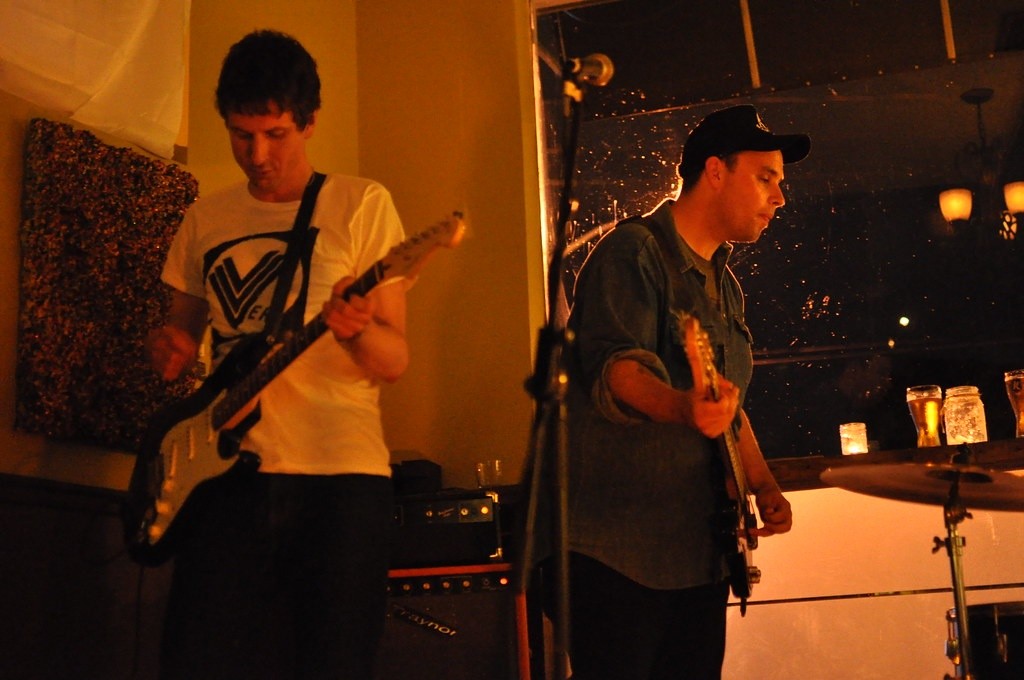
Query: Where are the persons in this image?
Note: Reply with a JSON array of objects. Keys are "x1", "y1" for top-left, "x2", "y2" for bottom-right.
[
  {"x1": 148, "y1": 29, "x2": 422, "y2": 679},
  {"x1": 542, "y1": 103, "x2": 809, "y2": 680}
]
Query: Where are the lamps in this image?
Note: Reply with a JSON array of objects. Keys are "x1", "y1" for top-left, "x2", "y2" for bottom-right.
[{"x1": 937, "y1": 86, "x2": 1024, "y2": 245}]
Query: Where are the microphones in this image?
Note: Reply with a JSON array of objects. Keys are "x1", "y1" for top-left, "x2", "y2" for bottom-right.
[{"x1": 563, "y1": 54, "x2": 614, "y2": 86}]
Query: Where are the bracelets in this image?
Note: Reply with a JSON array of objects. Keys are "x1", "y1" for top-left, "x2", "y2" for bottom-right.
[{"x1": 336, "y1": 324, "x2": 368, "y2": 342}]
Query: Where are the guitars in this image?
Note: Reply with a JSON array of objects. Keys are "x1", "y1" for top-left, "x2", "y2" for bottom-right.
[
  {"x1": 143, "y1": 208, "x2": 466, "y2": 568},
  {"x1": 666, "y1": 305, "x2": 761, "y2": 599}
]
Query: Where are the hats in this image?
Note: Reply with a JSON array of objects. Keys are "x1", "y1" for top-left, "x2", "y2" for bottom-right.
[{"x1": 683, "y1": 105, "x2": 813, "y2": 178}]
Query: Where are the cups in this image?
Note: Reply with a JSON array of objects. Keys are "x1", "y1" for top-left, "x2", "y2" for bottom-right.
[
  {"x1": 942, "y1": 386, "x2": 988, "y2": 445},
  {"x1": 471, "y1": 459, "x2": 503, "y2": 488},
  {"x1": 906, "y1": 384, "x2": 943, "y2": 447},
  {"x1": 838, "y1": 422, "x2": 869, "y2": 455},
  {"x1": 1005, "y1": 370, "x2": 1024, "y2": 438}
]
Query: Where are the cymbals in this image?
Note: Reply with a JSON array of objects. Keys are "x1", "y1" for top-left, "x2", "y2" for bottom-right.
[{"x1": 819, "y1": 461, "x2": 1024, "y2": 514}]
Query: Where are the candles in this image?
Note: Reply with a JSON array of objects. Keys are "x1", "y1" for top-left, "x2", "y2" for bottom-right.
[{"x1": 838, "y1": 422, "x2": 868, "y2": 456}]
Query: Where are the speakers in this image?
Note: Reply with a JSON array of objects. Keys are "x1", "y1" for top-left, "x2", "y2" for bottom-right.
[{"x1": 374, "y1": 563, "x2": 532, "y2": 680}]
